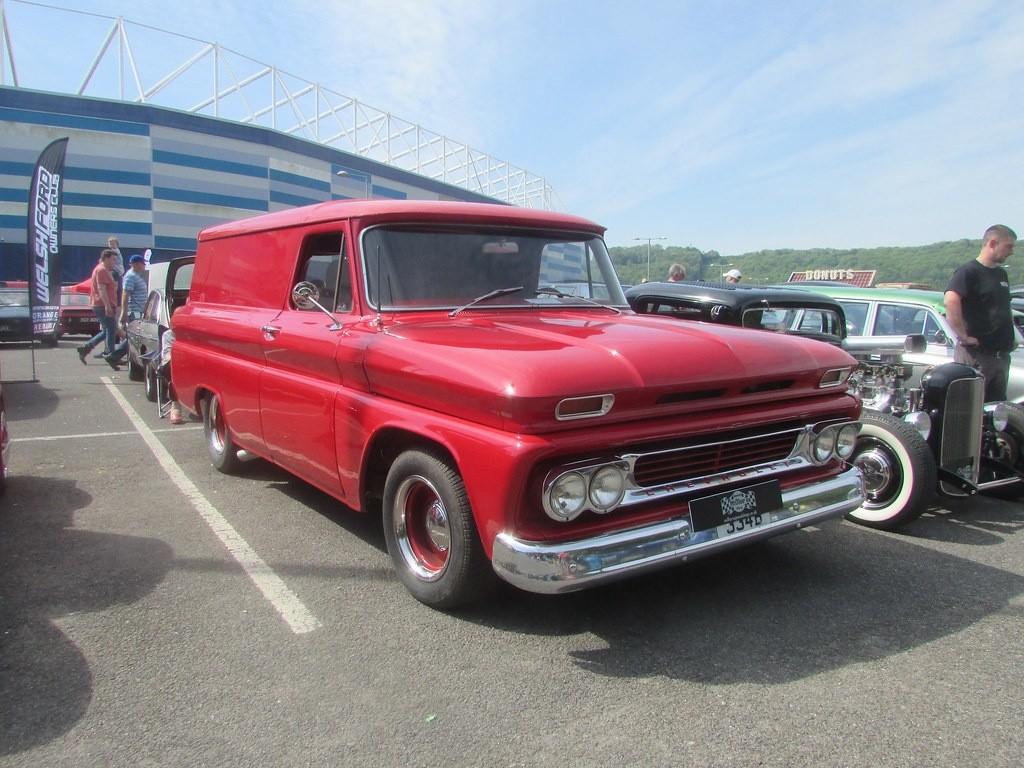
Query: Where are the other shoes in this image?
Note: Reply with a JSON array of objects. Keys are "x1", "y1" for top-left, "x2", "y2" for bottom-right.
[
  {"x1": 77, "y1": 346, "x2": 88, "y2": 364},
  {"x1": 170, "y1": 408, "x2": 182, "y2": 423},
  {"x1": 93, "y1": 351, "x2": 109, "y2": 358},
  {"x1": 117, "y1": 358, "x2": 127, "y2": 365},
  {"x1": 105, "y1": 356, "x2": 121, "y2": 370}
]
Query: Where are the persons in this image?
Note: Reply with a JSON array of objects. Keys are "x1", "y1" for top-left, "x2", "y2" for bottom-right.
[
  {"x1": 943, "y1": 224, "x2": 1017, "y2": 403},
  {"x1": 641, "y1": 278, "x2": 648, "y2": 283},
  {"x1": 76, "y1": 235, "x2": 149, "y2": 371},
  {"x1": 722, "y1": 269, "x2": 741, "y2": 283},
  {"x1": 667, "y1": 263, "x2": 686, "y2": 282},
  {"x1": 158, "y1": 330, "x2": 183, "y2": 424}
]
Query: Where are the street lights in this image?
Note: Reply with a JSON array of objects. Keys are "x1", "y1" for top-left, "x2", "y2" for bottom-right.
[
  {"x1": 710, "y1": 264, "x2": 735, "y2": 284},
  {"x1": 336, "y1": 171, "x2": 369, "y2": 199},
  {"x1": 749, "y1": 278, "x2": 769, "y2": 284},
  {"x1": 632, "y1": 236, "x2": 668, "y2": 281}
]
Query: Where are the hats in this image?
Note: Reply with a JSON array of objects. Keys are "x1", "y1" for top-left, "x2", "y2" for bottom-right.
[
  {"x1": 130, "y1": 255, "x2": 148, "y2": 263},
  {"x1": 723, "y1": 270, "x2": 742, "y2": 281}
]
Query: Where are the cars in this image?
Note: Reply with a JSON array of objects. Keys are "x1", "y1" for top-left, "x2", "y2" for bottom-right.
[
  {"x1": 126, "y1": 200, "x2": 1024, "y2": 607},
  {"x1": 0, "y1": 287, "x2": 32, "y2": 342},
  {"x1": 59, "y1": 293, "x2": 101, "y2": 339}
]
